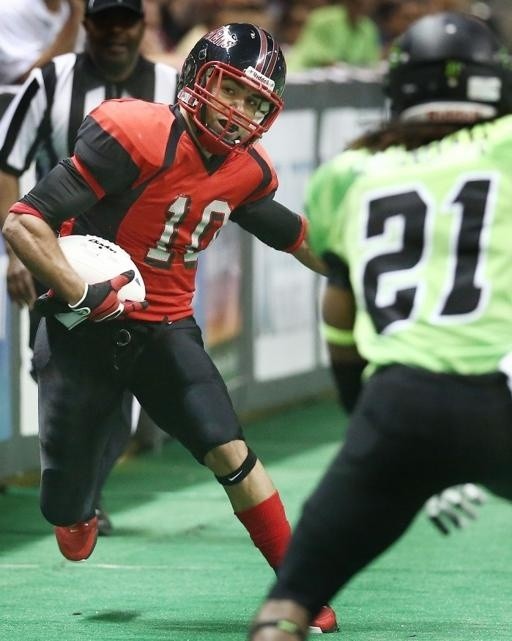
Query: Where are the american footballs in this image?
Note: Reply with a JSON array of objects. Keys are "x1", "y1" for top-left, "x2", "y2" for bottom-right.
[{"x1": 57, "y1": 234, "x2": 146, "y2": 303}]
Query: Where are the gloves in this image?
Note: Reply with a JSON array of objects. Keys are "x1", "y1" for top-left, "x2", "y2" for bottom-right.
[
  {"x1": 424, "y1": 483, "x2": 485, "y2": 536},
  {"x1": 68, "y1": 270, "x2": 149, "y2": 323}
]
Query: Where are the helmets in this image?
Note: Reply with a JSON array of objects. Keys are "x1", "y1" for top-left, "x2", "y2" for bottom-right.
[
  {"x1": 382, "y1": 11, "x2": 503, "y2": 120},
  {"x1": 177, "y1": 23, "x2": 287, "y2": 114},
  {"x1": 85, "y1": 0, "x2": 144, "y2": 17}
]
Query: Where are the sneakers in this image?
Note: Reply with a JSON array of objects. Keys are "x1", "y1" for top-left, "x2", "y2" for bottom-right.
[
  {"x1": 53, "y1": 506, "x2": 112, "y2": 562},
  {"x1": 248, "y1": 598, "x2": 338, "y2": 641}
]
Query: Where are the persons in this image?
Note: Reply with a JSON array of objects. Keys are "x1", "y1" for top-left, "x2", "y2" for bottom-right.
[
  {"x1": 0, "y1": 0, "x2": 511, "y2": 640},
  {"x1": 1, "y1": 2, "x2": 87, "y2": 85},
  {"x1": 249, "y1": 11, "x2": 508, "y2": 641},
  {"x1": 3, "y1": 22, "x2": 338, "y2": 635},
  {"x1": 141, "y1": 1, "x2": 435, "y2": 78}
]
